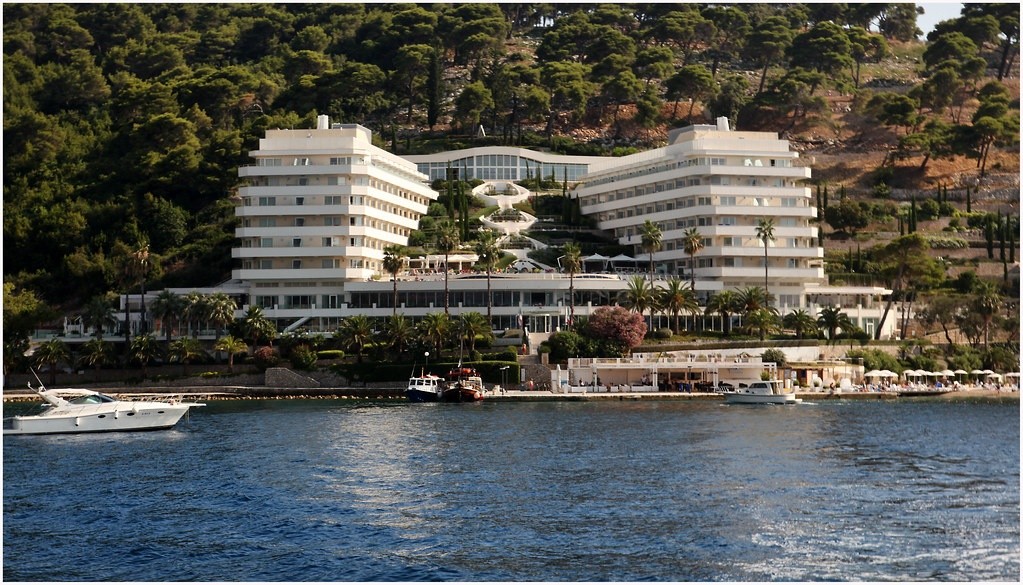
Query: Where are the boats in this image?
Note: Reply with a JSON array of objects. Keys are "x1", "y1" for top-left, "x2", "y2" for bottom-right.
[
  {"x1": 716, "y1": 381, "x2": 803, "y2": 404},
  {"x1": 404, "y1": 365, "x2": 446, "y2": 401},
  {"x1": 444, "y1": 336, "x2": 486, "y2": 402},
  {"x1": 12, "y1": 367, "x2": 206, "y2": 432}
]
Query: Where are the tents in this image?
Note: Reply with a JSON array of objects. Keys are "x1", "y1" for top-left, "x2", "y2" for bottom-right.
[{"x1": 582, "y1": 253, "x2": 636, "y2": 273}]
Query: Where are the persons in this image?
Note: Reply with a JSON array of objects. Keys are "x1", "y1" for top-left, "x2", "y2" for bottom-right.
[
  {"x1": 936, "y1": 380, "x2": 942, "y2": 387},
  {"x1": 861, "y1": 378, "x2": 882, "y2": 392},
  {"x1": 521, "y1": 343, "x2": 526, "y2": 356},
  {"x1": 641, "y1": 375, "x2": 649, "y2": 385},
  {"x1": 529, "y1": 378, "x2": 534, "y2": 391}
]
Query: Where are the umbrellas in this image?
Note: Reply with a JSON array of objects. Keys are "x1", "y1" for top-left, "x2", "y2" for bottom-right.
[
  {"x1": 987, "y1": 372, "x2": 1020, "y2": 385},
  {"x1": 863, "y1": 369, "x2": 899, "y2": 384},
  {"x1": 903, "y1": 369, "x2": 955, "y2": 385},
  {"x1": 982, "y1": 370, "x2": 994, "y2": 374},
  {"x1": 971, "y1": 369, "x2": 983, "y2": 385},
  {"x1": 954, "y1": 369, "x2": 967, "y2": 382}
]
